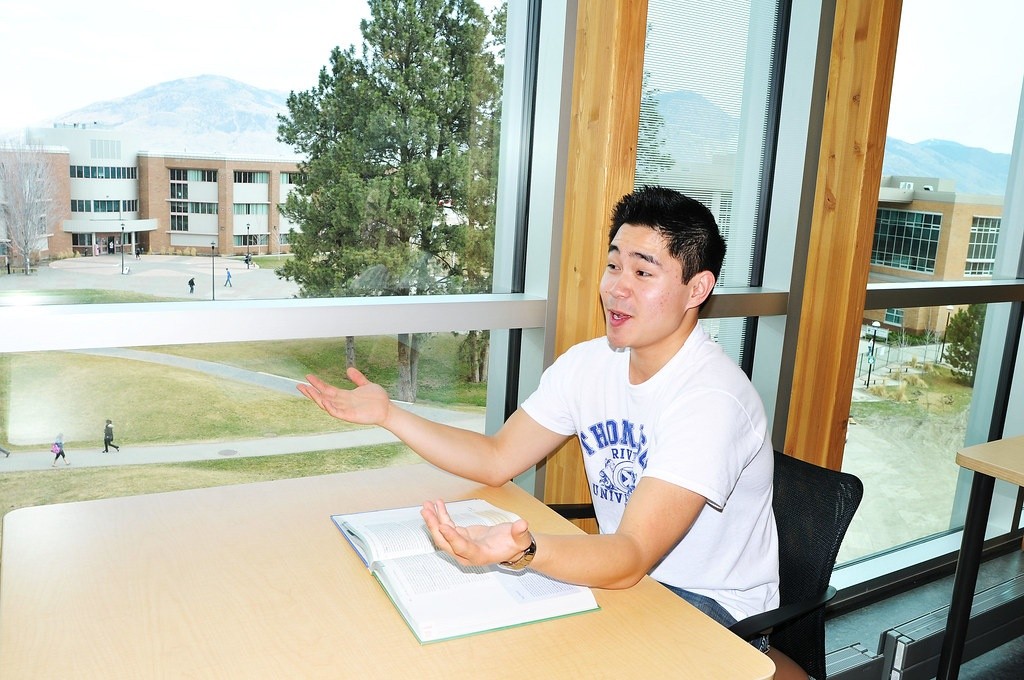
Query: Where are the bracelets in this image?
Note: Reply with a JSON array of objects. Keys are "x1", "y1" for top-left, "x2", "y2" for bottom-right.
[{"x1": 497, "y1": 531, "x2": 537, "y2": 571}]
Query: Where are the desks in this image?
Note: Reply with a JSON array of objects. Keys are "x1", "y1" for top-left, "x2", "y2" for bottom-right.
[
  {"x1": 0, "y1": 454, "x2": 784, "y2": 679},
  {"x1": 936, "y1": 435, "x2": 1024, "y2": 680}
]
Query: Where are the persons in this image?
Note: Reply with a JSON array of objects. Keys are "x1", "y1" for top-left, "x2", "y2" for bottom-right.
[
  {"x1": 52, "y1": 433, "x2": 70, "y2": 466},
  {"x1": 296, "y1": 184, "x2": 779, "y2": 653},
  {"x1": 224, "y1": 268, "x2": 232, "y2": 287},
  {"x1": 245, "y1": 252, "x2": 251, "y2": 264},
  {"x1": 103, "y1": 420, "x2": 119, "y2": 453},
  {"x1": 109, "y1": 240, "x2": 114, "y2": 254},
  {"x1": 136, "y1": 246, "x2": 143, "y2": 258},
  {"x1": 0, "y1": 447, "x2": 10, "y2": 457},
  {"x1": 867, "y1": 338, "x2": 873, "y2": 356},
  {"x1": 279, "y1": 268, "x2": 289, "y2": 281},
  {"x1": 188, "y1": 278, "x2": 195, "y2": 293}
]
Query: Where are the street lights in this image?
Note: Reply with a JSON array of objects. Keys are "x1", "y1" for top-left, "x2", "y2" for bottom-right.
[
  {"x1": 247, "y1": 224, "x2": 250, "y2": 270},
  {"x1": 940, "y1": 305, "x2": 954, "y2": 362},
  {"x1": 211, "y1": 241, "x2": 216, "y2": 301},
  {"x1": 866, "y1": 321, "x2": 881, "y2": 388},
  {"x1": 121, "y1": 223, "x2": 125, "y2": 274}
]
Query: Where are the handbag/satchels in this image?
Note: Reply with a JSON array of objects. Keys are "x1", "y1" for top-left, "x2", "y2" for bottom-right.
[{"x1": 51, "y1": 443, "x2": 61, "y2": 454}]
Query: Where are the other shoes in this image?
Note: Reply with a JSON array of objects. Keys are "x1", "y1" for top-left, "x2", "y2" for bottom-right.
[
  {"x1": 117, "y1": 446, "x2": 119, "y2": 452},
  {"x1": 67, "y1": 462, "x2": 70, "y2": 465},
  {"x1": 52, "y1": 464, "x2": 57, "y2": 467},
  {"x1": 103, "y1": 450, "x2": 108, "y2": 454},
  {"x1": 6, "y1": 452, "x2": 10, "y2": 458}
]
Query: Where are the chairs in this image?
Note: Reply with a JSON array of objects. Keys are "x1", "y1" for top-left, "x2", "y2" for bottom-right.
[{"x1": 547, "y1": 448, "x2": 869, "y2": 680}]
